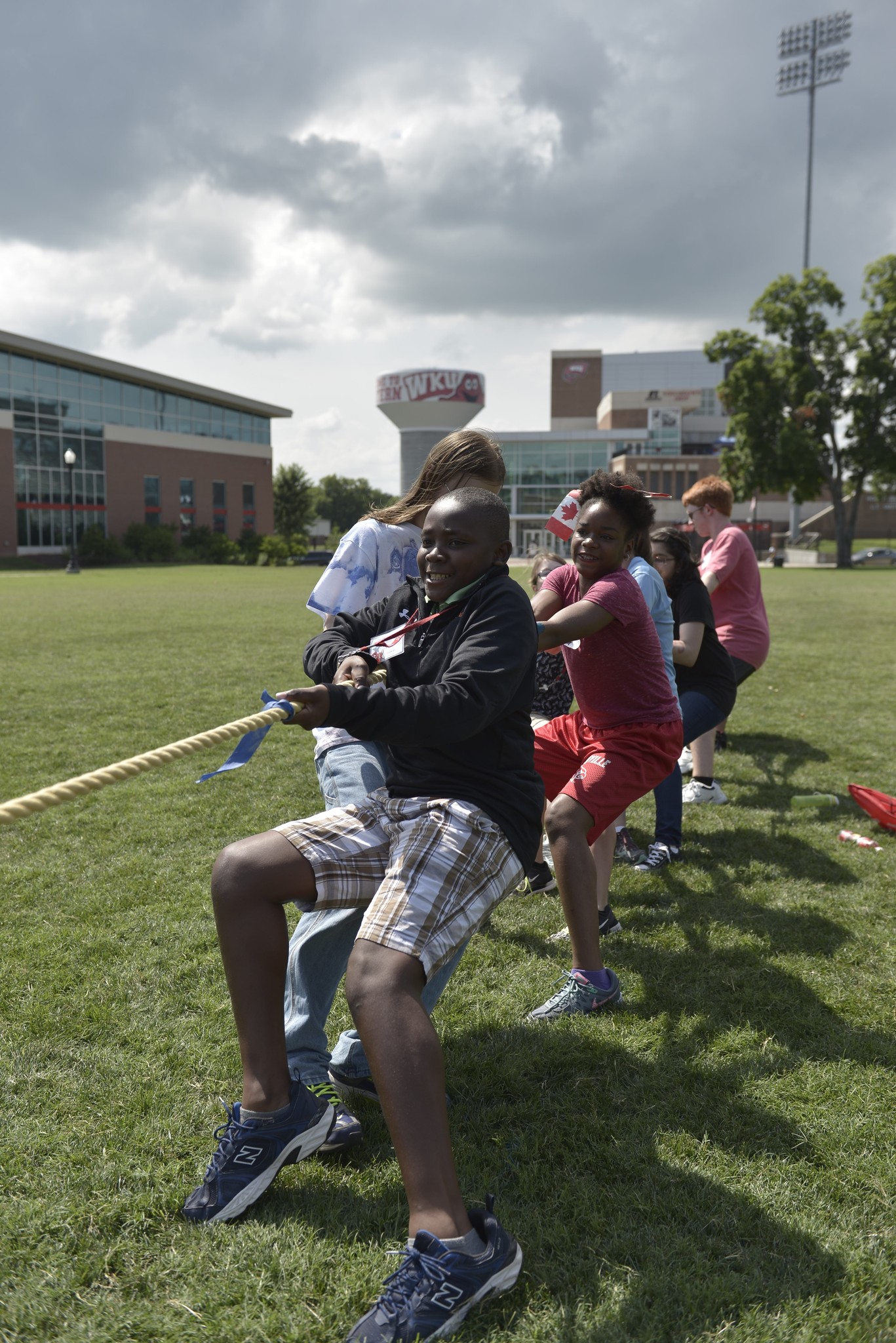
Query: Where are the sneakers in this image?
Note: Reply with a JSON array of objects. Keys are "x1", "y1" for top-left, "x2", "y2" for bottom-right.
[
  {"x1": 714, "y1": 730, "x2": 727, "y2": 750},
  {"x1": 181, "y1": 1065, "x2": 336, "y2": 1223},
  {"x1": 546, "y1": 904, "x2": 623, "y2": 943},
  {"x1": 508, "y1": 861, "x2": 556, "y2": 900},
  {"x1": 614, "y1": 825, "x2": 647, "y2": 865},
  {"x1": 328, "y1": 1062, "x2": 452, "y2": 1112},
  {"x1": 682, "y1": 778, "x2": 727, "y2": 804},
  {"x1": 299, "y1": 1081, "x2": 363, "y2": 1152},
  {"x1": 526, "y1": 968, "x2": 621, "y2": 1021},
  {"x1": 345, "y1": 1193, "x2": 523, "y2": 1343},
  {"x1": 632, "y1": 841, "x2": 683, "y2": 872},
  {"x1": 677, "y1": 746, "x2": 696, "y2": 774},
  {"x1": 542, "y1": 835, "x2": 556, "y2": 871}
]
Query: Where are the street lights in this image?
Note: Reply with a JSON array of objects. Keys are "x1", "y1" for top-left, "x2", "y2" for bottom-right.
[
  {"x1": 64, "y1": 448, "x2": 79, "y2": 573},
  {"x1": 773, "y1": 10, "x2": 851, "y2": 544}
]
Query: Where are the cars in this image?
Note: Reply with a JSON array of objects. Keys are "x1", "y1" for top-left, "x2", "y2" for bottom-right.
[
  {"x1": 296, "y1": 551, "x2": 333, "y2": 566},
  {"x1": 851, "y1": 546, "x2": 896, "y2": 567}
]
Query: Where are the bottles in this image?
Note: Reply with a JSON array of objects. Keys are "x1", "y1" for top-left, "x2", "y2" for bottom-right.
[
  {"x1": 838, "y1": 829, "x2": 884, "y2": 852},
  {"x1": 790, "y1": 791, "x2": 839, "y2": 809}
]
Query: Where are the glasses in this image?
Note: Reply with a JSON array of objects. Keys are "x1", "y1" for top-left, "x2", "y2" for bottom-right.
[
  {"x1": 652, "y1": 557, "x2": 675, "y2": 564},
  {"x1": 533, "y1": 569, "x2": 552, "y2": 585},
  {"x1": 687, "y1": 504, "x2": 715, "y2": 519}
]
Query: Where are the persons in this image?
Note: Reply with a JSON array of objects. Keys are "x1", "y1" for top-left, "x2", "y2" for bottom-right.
[{"x1": 182, "y1": 427, "x2": 775, "y2": 1343}]
